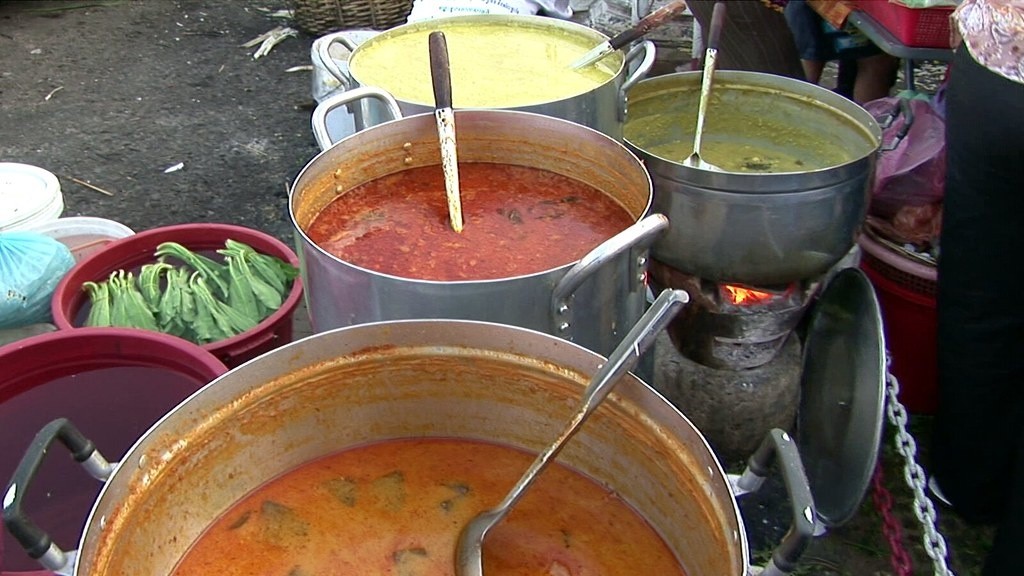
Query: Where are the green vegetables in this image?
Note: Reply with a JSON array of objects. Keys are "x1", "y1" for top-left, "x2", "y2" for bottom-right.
[{"x1": 80, "y1": 239, "x2": 301, "y2": 345}]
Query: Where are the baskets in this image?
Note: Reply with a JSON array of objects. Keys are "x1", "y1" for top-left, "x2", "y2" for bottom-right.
[
  {"x1": 858, "y1": 0, "x2": 956, "y2": 48},
  {"x1": 292, "y1": 0, "x2": 414, "y2": 37}
]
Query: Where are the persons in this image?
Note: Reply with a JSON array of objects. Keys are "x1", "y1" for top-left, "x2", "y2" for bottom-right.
[
  {"x1": 928, "y1": 0, "x2": 1024, "y2": 576},
  {"x1": 690, "y1": 17, "x2": 704, "y2": 70},
  {"x1": 783, "y1": 0, "x2": 896, "y2": 107}
]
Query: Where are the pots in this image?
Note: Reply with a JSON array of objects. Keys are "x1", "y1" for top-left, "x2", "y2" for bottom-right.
[
  {"x1": 285, "y1": 85, "x2": 671, "y2": 389},
  {"x1": 0, "y1": 318, "x2": 817, "y2": 576},
  {"x1": 620, "y1": 69, "x2": 913, "y2": 288},
  {"x1": 317, "y1": 13, "x2": 657, "y2": 145}
]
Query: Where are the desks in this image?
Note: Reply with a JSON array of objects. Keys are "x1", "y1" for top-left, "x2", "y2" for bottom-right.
[{"x1": 845, "y1": 10, "x2": 957, "y2": 95}]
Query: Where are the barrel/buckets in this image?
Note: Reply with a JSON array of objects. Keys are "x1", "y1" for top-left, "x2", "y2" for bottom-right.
[
  {"x1": 0, "y1": 160, "x2": 64, "y2": 234},
  {"x1": 50, "y1": 222, "x2": 304, "y2": 371},
  {"x1": 19, "y1": 216, "x2": 136, "y2": 266},
  {"x1": 0, "y1": 326, "x2": 229, "y2": 576}
]
